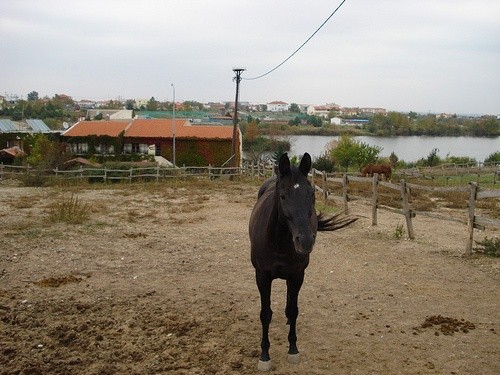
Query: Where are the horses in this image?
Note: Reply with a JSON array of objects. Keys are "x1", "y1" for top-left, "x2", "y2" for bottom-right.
[
  {"x1": 360, "y1": 164, "x2": 381, "y2": 179},
  {"x1": 377, "y1": 162, "x2": 392, "y2": 183},
  {"x1": 249, "y1": 151, "x2": 360, "y2": 372}
]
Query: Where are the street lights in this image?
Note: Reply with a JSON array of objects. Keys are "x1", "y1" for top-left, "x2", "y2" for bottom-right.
[{"x1": 230, "y1": 69, "x2": 246, "y2": 181}]
